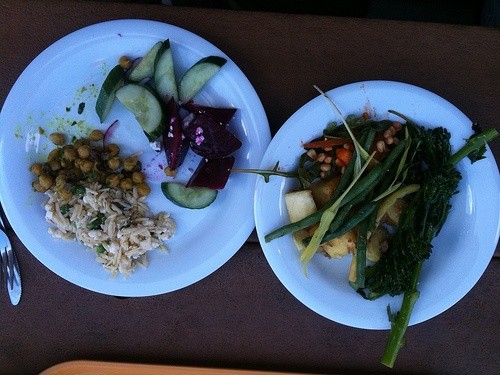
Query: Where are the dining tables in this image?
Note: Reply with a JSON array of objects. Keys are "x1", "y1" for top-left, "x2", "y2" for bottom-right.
[{"x1": 0, "y1": 0, "x2": 500, "y2": 375}]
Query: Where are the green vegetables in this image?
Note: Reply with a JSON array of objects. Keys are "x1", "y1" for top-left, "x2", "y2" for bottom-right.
[{"x1": 356, "y1": 124, "x2": 500, "y2": 369}]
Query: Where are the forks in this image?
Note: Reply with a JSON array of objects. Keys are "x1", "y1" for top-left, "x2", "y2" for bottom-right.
[{"x1": 0, "y1": 229, "x2": 14, "y2": 291}]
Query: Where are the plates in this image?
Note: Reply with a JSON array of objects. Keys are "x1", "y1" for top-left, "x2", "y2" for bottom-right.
[
  {"x1": 254, "y1": 80, "x2": 500, "y2": 330},
  {"x1": 0, "y1": 20, "x2": 272, "y2": 298},
  {"x1": 39, "y1": 360, "x2": 312, "y2": 375}
]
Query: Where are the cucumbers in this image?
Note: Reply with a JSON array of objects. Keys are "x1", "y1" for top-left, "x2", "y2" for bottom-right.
[
  {"x1": 95, "y1": 38, "x2": 227, "y2": 141},
  {"x1": 161, "y1": 182, "x2": 218, "y2": 209}
]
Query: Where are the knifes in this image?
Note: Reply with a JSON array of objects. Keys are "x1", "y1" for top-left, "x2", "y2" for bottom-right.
[{"x1": 0, "y1": 214, "x2": 23, "y2": 306}]
